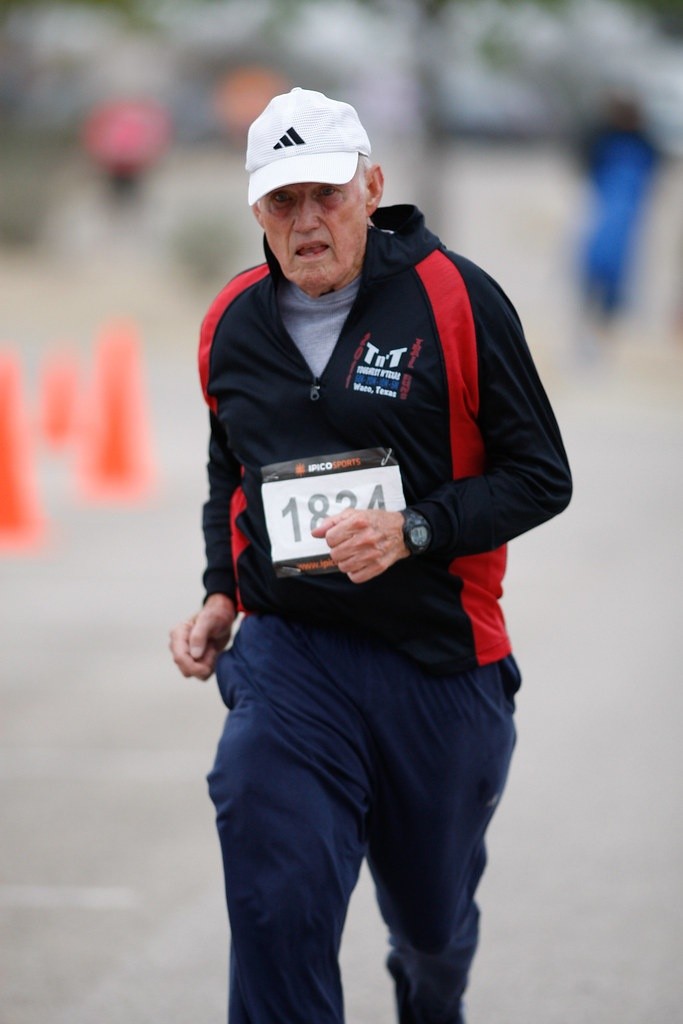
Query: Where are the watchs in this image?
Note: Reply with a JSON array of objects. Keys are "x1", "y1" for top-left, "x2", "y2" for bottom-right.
[{"x1": 399, "y1": 508, "x2": 433, "y2": 556}]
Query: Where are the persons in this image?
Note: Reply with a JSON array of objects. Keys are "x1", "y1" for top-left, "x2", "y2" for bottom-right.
[
  {"x1": 573, "y1": 93, "x2": 662, "y2": 334},
  {"x1": 85, "y1": 90, "x2": 175, "y2": 210},
  {"x1": 211, "y1": 56, "x2": 279, "y2": 149},
  {"x1": 169, "y1": 86, "x2": 573, "y2": 1024}
]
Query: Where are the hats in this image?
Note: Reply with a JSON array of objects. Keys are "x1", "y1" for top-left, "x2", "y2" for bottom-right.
[{"x1": 244, "y1": 89, "x2": 369, "y2": 206}]
